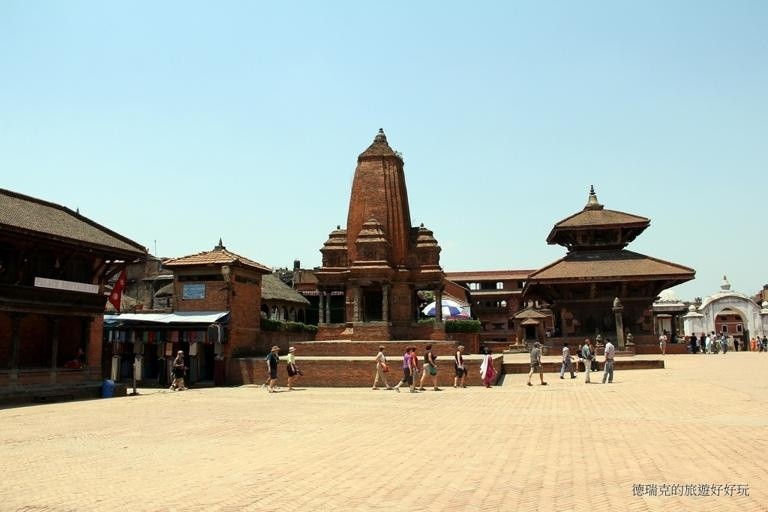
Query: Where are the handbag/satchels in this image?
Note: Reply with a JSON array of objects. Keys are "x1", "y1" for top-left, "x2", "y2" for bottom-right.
[
  {"x1": 430, "y1": 366, "x2": 436, "y2": 376},
  {"x1": 383, "y1": 364, "x2": 389, "y2": 372}
]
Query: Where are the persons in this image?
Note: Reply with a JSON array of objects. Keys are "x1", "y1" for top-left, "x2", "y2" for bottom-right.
[
  {"x1": 266, "y1": 345, "x2": 280, "y2": 393},
  {"x1": 559, "y1": 342, "x2": 576, "y2": 379},
  {"x1": 419, "y1": 344, "x2": 441, "y2": 391},
  {"x1": 575, "y1": 344, "x2": 586, "y2": 372},
  {"x1": 674, "y1": 331, "x2": 767, "y2": 355},
  {"x1": 394, "y1": 346, "x2": 417, "y2": 393},
  {"x1": 410, "y1": 347, "x2": 419, "y2": 390},
  {"x1": 286, "y1": 347, "x2": 300, "y2": 390},
  {"x1": 659, "y1": 331, "x2": 667, "y2": 354},
  {"x1": 169, "y1": 363, "x2": 189, "y2": 389},
  {"x1": 372, "y1": 344, "x2": 393, "y2": 390},
  {"x1": 259, "y1": 352, "x2": 279, "y2": 389},
  {"x1": 454, "y1": 345, "x2": 466, "y2": 388},
  {"x1": 602, "y1": 338, "x2": 614, "y2": 384},
  {"x1": 479, "y1": 349, "x2": 498, "y2": 388},
  {"x1": 591, "y1": 348, "x2": 597, "y2": 371},
  {"x1": 582, "y1": 339, "x2": 592, "y2": 383},
  {"x1": 174, "y1": 350, "x2": 188, "y2": 391},
  {"x1": 527, "y1": 342, "x2": 547, "y2": 386}
]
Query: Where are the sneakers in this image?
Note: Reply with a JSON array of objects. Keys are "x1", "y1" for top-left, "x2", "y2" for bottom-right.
[
  {"x1": 371, "y1": 387, "x2": 380, "y2": 390},
  {"x1": 266, "y1": 385, "x2": 281, "y2": 393},
  {"x1": 409, "y1": 386, "x2": 426, "y2": 393},
  {"x1": 527, "y1": 383, "x2": 533, "y2": 386},
  {"x1": 387, "y1": 387, "x2": 393, "y2": 390},
  {"x1": 394, "y1": 386, "x2": 401, "y2": 393},
  {"x1": 541, "y1": 382, "x2": 547, "y2": 385},
  {"x1": 454, "y1": 384, "x2": 466, "y2": 388},
  {"x1": 434, "y1": 388, "x2": 442, "y2": 391}
]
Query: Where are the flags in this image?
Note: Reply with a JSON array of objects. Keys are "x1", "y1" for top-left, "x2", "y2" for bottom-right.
[{"x1": 109, "y1": 267, "x2": 126, "y2": 313}]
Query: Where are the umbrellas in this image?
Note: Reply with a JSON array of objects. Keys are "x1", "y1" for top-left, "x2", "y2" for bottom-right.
[{"x1": 420, "y1": 300, "x2": 463, "y2": 317}]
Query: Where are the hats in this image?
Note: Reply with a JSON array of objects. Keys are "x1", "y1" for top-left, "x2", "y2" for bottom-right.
[
  {"x1": 534, "y1": 341, "x2": 542, "y2": 346},
  {"x1": 271, "y1": 345, "x2": 280, "y2": 352},
  {"x1": 289, "y1": 346, "x2": 296, "y2": 353}
]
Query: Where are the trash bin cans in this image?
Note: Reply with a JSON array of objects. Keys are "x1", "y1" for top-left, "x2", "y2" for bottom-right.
[{"x1": 102, "y1": 380, "x2": 114, "y2": 398}]
